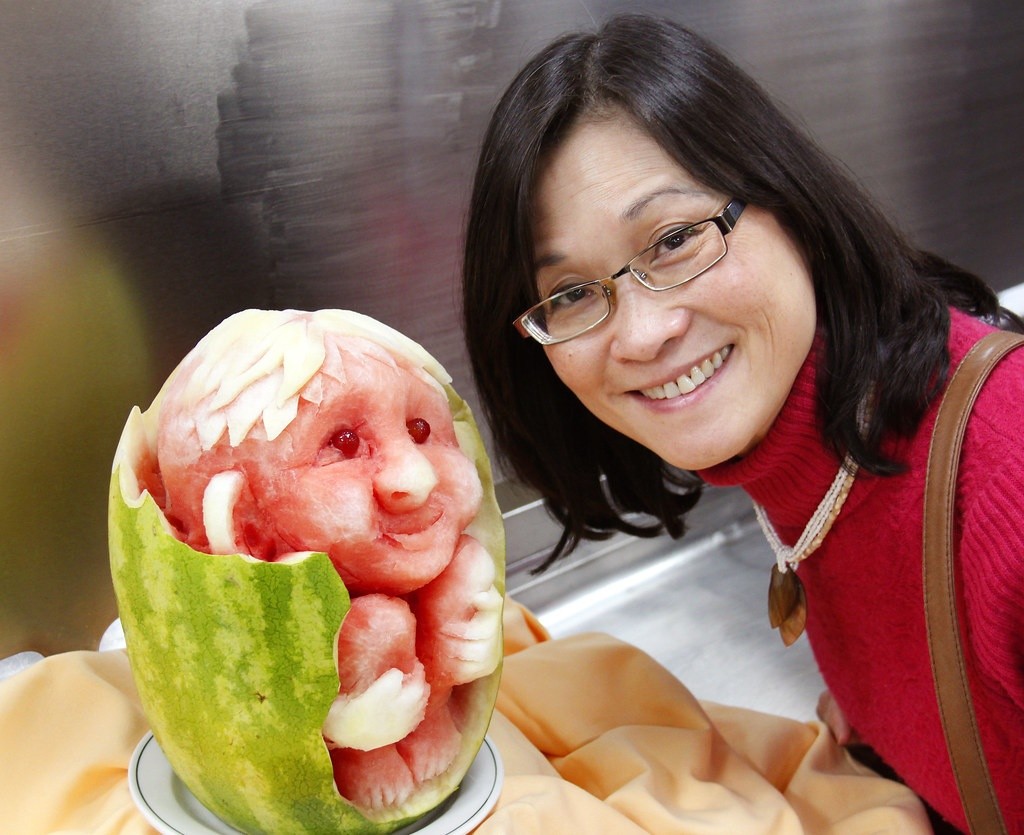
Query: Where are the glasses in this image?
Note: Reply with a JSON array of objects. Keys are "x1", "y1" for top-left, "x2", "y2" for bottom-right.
[{"x1": 512, "y1": 196, "x2": 749, "y2": 345}]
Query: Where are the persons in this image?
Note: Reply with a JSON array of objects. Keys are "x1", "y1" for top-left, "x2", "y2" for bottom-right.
[{"x1": 464, "y1": 13, "x2": 1024, "y2": 835}]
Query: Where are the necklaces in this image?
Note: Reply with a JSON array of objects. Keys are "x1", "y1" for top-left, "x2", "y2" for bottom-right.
[{"x1": 753, "y1": 400, "x2": 873, "y2": 642}]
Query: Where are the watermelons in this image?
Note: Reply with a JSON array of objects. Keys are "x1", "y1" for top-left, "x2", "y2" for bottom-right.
[{"x1": 107, "y1": 308, "x2": 509, "y2": 835}]
